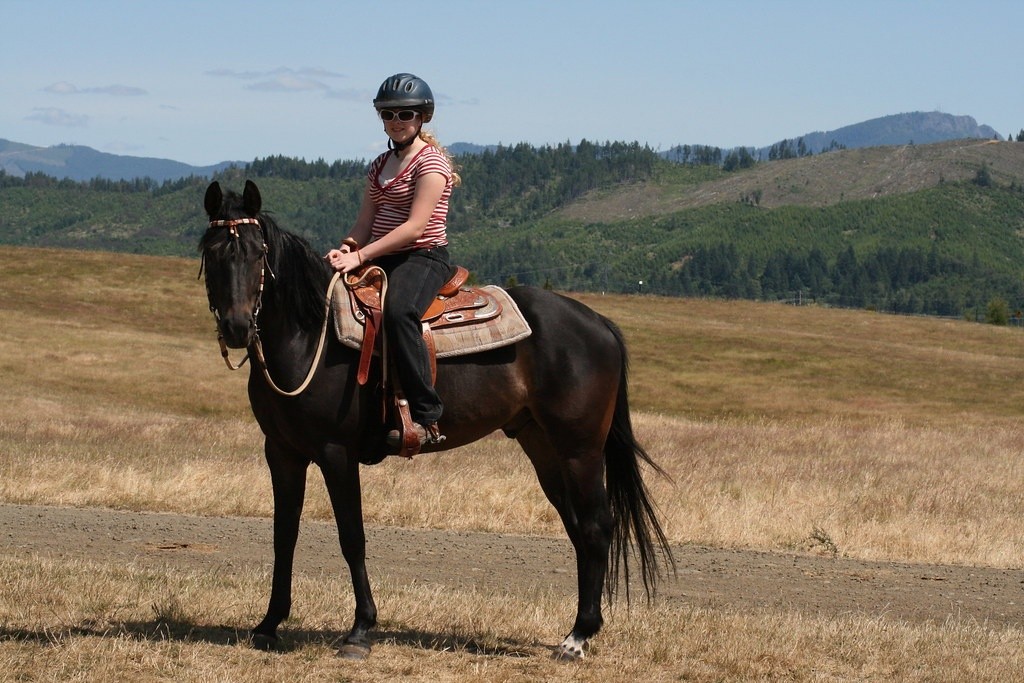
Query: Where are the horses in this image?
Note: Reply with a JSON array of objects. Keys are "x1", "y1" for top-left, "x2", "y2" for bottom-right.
[{"x1": 197, "y1": 179, "x2": 679, "y2": 666}]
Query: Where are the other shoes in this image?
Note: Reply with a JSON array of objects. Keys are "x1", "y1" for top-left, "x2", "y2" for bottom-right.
[{"x1": 387, "y1": 421, "x2": 440, "y2": 446}]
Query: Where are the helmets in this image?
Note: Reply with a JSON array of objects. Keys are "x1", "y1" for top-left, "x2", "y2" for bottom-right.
[{"x1": 373, "y1": 73, "x2": 434, "y2": 123}]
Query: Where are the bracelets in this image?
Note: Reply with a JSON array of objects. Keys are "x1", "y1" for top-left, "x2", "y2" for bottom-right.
[{"x1": 357, "y1": 250, "x2": 362, "y2": 266}]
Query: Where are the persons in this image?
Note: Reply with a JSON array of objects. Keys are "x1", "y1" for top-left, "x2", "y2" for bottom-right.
[{"x1": 324, "y1": 73, "x2": 462, "y2": 446}]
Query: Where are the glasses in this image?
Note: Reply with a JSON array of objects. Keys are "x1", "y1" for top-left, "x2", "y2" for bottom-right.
[{"x1": 379, "y1": 109, "x2": 418, "y2": 122}]
